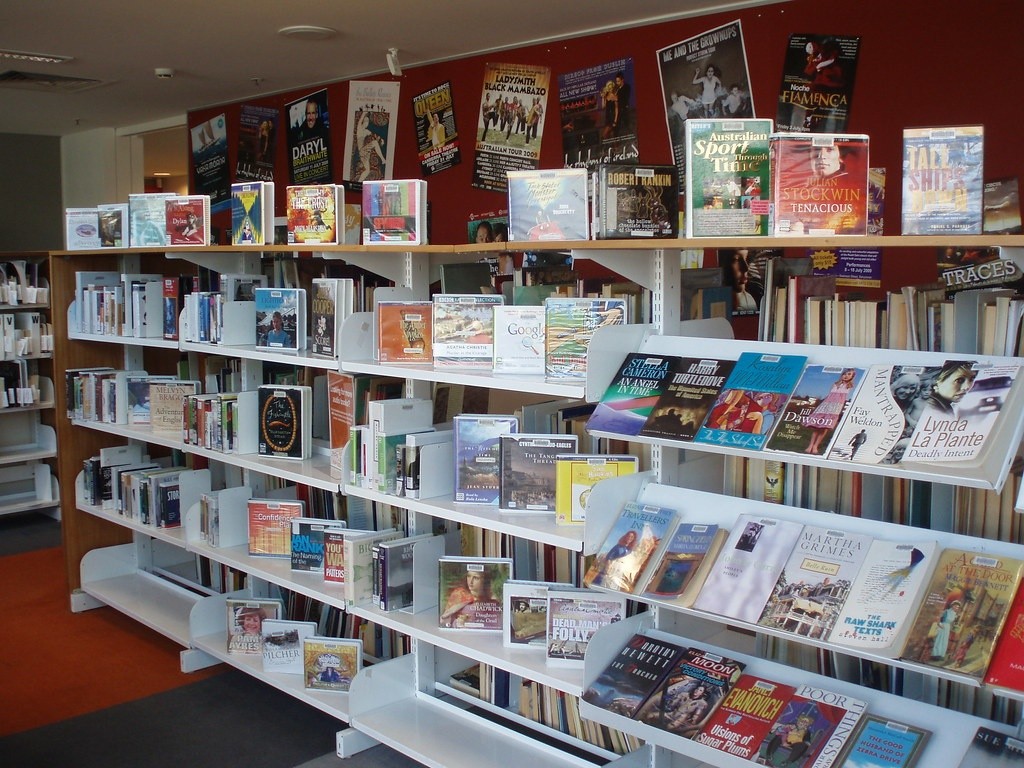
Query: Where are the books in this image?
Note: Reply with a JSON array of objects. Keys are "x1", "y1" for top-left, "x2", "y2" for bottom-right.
[{"x1": 63, "y1": 120, "x2": 1024, "y2": 768}]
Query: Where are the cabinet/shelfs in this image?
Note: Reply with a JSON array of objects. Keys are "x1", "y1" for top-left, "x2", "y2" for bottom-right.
[{"x1": 1, "y1": 235, "x2": 1023, "y2": 768}]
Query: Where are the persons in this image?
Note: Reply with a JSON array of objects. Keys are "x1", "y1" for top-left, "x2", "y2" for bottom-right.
[
  {"x1": 671, "y1": 65, "x2": 746, "y2": 120},
  {"x1": 810, "y1": 144, "x2": 848, "y2": 178},
  {"x1": 299, "y1": 100, "x2": 323, "y2": 138},
  {"x1": 269, "y1": 119, "x2": 275, "y2": 141},
  {"x1": 718, "y1": 246, "x2": 759, "y2": 319},
  {"x1": 931, "y1": 360, "x2": 978, "y2": 416},
  {"x1": 904, "y1": 378, "x2": 934, "y2": 437},
  {"x1": 476, "y1": 221, "x2": 492, "y2": 243},
  {"x1": 267, "y1": 311, "x2": 292, "y2": 348},
  {"x1": 890, "y1": 373, "x2": 920, "y2": 427},
  {"x1": 491, "y1": 222, "x2": 508, "y2": 243},
  {"x1": 229, "y1": 607, "x2": 267, "y2": 653},
  {"x1": 515, "y1": 598, "x2": 530, "y2": 614},
  {"x1": 601, "y1": 72, "x2": 626, "y2": 139},
  {"x1": 481, "y1": 93, "x2": 543, "y2": 147},
  {"x1": 802, "y1": 368, "x2": 856, "y2": 456},
  {"x1": 425, "y1": 103, "x2": 445, "y2": 149},
  {"x1": 256, "y1": 120, "x2": 270, "y2": 161}
]
